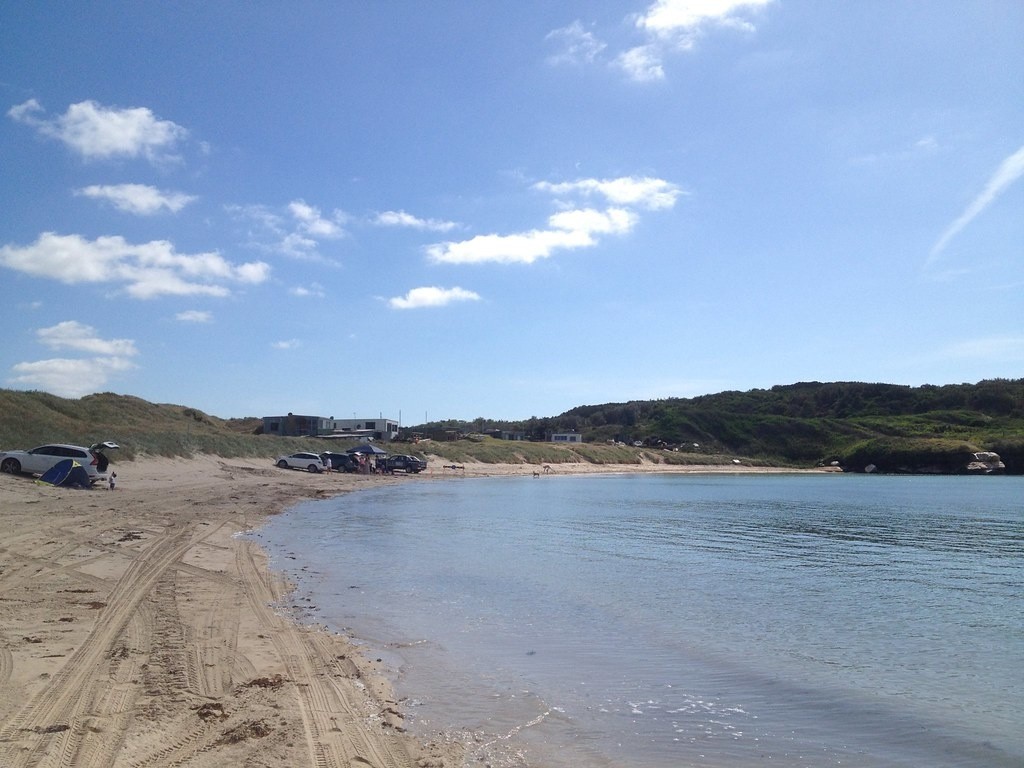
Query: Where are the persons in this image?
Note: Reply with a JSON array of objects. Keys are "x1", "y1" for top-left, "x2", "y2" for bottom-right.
[
  {"x1": 353, "y1": 454, "x2": 372, "y2": 473},
  {"x1": 375, "y1": 455, "x2": 387, "y2": 473},
  {"x1": 324, "y1": 456, "x2": 333, "y2": 476}
]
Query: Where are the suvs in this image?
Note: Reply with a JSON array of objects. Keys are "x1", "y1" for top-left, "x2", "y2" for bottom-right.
[{"x1": 0, "y1": 442, "x2": 120, "y2": 487}]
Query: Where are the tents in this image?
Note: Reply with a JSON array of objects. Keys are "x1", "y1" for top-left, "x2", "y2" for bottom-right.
[{"x1": 345, "y1": 443, "x2": 389, "y2": 476}]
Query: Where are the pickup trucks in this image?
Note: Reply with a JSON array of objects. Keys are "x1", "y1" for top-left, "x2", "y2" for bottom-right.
[{"x1": 376, "y1": 455, "x2": 427, "y2": 473}]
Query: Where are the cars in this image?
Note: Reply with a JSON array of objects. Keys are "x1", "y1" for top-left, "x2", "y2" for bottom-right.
[{"x1": 275, "y1": 453, "x2": 352, "y2": 473}]
